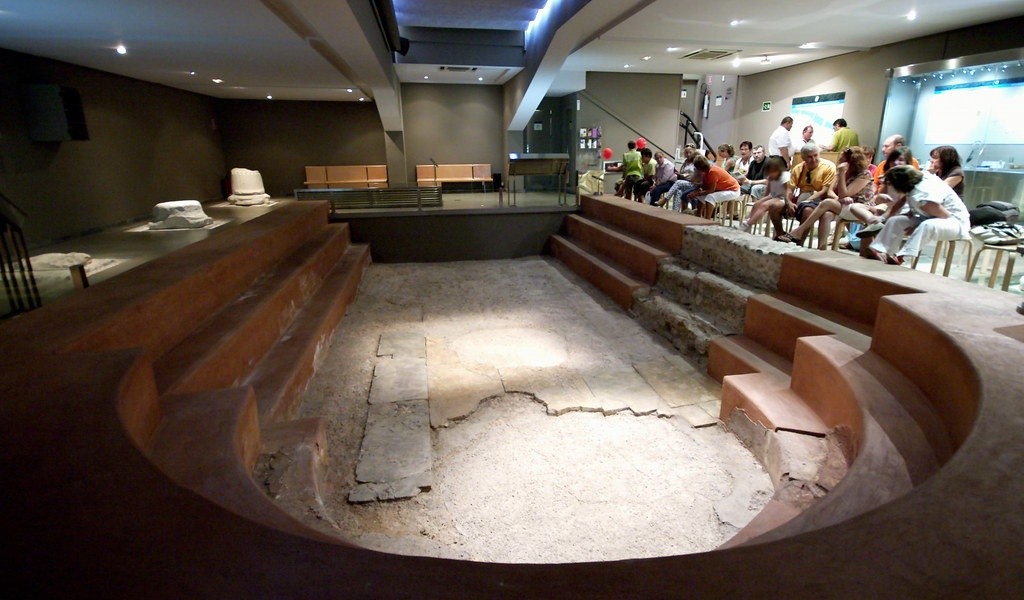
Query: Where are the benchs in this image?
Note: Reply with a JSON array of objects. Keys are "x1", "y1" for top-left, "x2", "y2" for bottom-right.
[
  {"x1": 415, "y1": 164, "x2": 494, "y2": 192},
  {"x1": 304, "y1": 165, "x2": 388, "y2": 188}
]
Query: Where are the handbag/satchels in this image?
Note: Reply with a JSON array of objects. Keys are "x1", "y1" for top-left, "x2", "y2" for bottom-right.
[
  {"x1": 971, "y1": 221, "x2": 1024, "y2": 245},
  {"x1": 969, "y1": 200, "x2": 1020, "y2": 227}
]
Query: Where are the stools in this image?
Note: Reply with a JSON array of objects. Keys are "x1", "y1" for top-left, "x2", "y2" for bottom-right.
[
  {"x1": 967, "y1": 243, "x2": 1024, "y2": 292},
  {"x1": 912, "y1": 237, "x2": 974, "y2": 277},
  {"x1": 714, "y1": 202, "x2": 865, "y2": 250}
]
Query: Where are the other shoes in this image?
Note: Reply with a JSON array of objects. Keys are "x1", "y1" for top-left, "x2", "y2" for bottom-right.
[
  {"x1": 726, "y1": 215, "x2": 738, "y2": 220},
  {"x1": 734, "y1": 220, "x2": 752, "y2": 234},
  {"x1": 866, "y1": 246, "x2": 886, "y2": 264},
  {"x1": 855, "y1": 222, "x2": 884, "y2": 238},
  {"x1": 887, "y1": 255, "x2": 904, "y2": 265},
  {"x1": 655, "y1": 200, "x2": 665, "y2": 208}
]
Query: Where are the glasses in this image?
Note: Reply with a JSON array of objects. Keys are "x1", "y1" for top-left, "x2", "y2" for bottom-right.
[
  {"x1": 685, "y1": 145, "x2": 695, "y2": 148},
  {"x1": 806, "y1": 170, "x2": 811, "y2": 184}
]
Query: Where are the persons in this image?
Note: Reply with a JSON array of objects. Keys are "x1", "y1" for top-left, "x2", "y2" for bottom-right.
[{"x1": 607, "y1": 116, "x2": 971, "y2": 267}]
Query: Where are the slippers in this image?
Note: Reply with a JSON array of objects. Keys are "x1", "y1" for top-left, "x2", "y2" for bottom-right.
[
  {"x1": 776, "y1": 232, "x2": 801, "y2": 244},
  {"x1": 774, "y1": 230, "x2": 786, "y2": 242}
]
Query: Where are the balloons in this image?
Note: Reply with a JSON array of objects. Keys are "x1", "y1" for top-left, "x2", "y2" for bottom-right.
[
  {"x1": 601, "y1": 147, "x2": 612, "y2": 159},
  {"x1": 636, "y1": 137, "x2": 647, "y2": 149}
]
